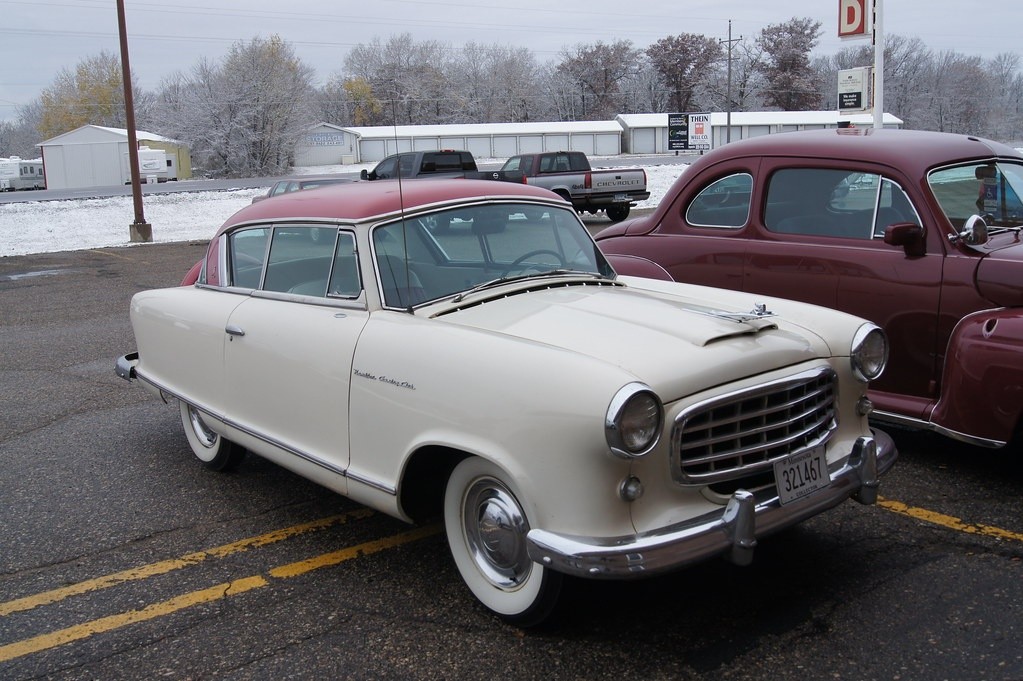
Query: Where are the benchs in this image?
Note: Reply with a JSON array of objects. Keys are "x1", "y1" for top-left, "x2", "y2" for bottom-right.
[
  {"x1": 235, "y1": 254, "x2": 356, "y2": 291},
  {"x1": 688, "y1": 202, "x2": 790, "y2": 225}
]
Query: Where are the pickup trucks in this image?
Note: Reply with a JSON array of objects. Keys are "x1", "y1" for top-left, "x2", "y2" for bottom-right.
[
  {"x1": 360, "y1": 149, "x2": 528, "y2": 185},
  {"x1": 500, "y1": 151, "x2": 650, "y2": 222}
]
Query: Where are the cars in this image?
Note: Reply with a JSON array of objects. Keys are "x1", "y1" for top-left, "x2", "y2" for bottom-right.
[
  {"x1": 593, "y1": 127, "x2": 1023, "y2": 449},
  {"x1": 115, "y1": 178, "x2": 900, "y2": 629},
  {"x1": 251, "y1": 179, "x2": 354, "y2": 203}
]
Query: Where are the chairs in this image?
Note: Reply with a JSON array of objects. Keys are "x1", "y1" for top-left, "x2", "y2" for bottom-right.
[
  {"x1": 860, "y1": 206, "x2": 904, "y2": 235},
  {"x1": 778, "y1": 214, "x2": 842, "y2": 235},
  {"x1": 556, "y1": 163, "x2": 568, "y2": 171},
  {"x1": 377, "y1": 255, "x2": 425, "y2": 307}
]
